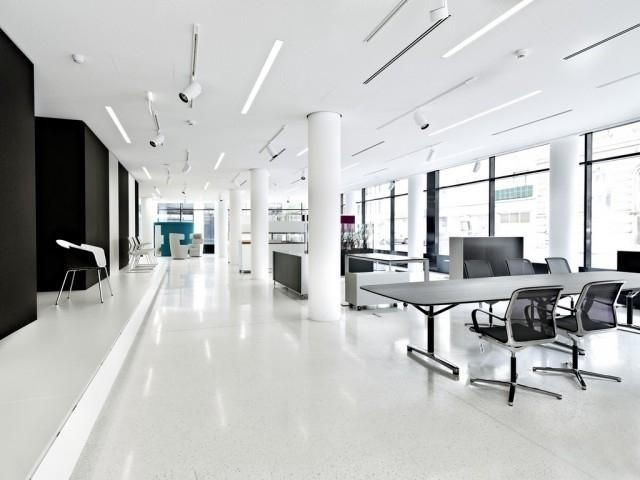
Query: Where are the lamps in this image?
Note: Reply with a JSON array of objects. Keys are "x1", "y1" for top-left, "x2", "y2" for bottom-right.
[
  {"x1": 176, "y1": 82, "x2": 204, "y2": 103},
  {"x1": 150, "y1": 134, "x2": 164, "y2": 149}
]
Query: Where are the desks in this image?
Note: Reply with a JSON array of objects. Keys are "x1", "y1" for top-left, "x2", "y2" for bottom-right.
[
  {"x1": 356, "y1": 269, "x2": 639, "y2": 380},
  {"x1": 345, "y1": 252, "x2": 430, "y2": 282}
]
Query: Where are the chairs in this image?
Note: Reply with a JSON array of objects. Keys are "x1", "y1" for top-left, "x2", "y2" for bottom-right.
[
  {"x1": 127, "y1": 236, "x2": 157, "y2": 273},
  {"x1": 464, "y1": 252, "x2": 639, "y2": 406},
  {"x1": 167, "y1": 233, "x2": 204, "y2": 258},
  {"x1": 55, "y1": 238, "x2": 114, "y2": 307}
]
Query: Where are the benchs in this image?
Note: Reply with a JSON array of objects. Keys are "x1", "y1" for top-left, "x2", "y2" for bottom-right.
[{"x1": 345, "y1": 270, "x2": 409, "y2": 310}]
